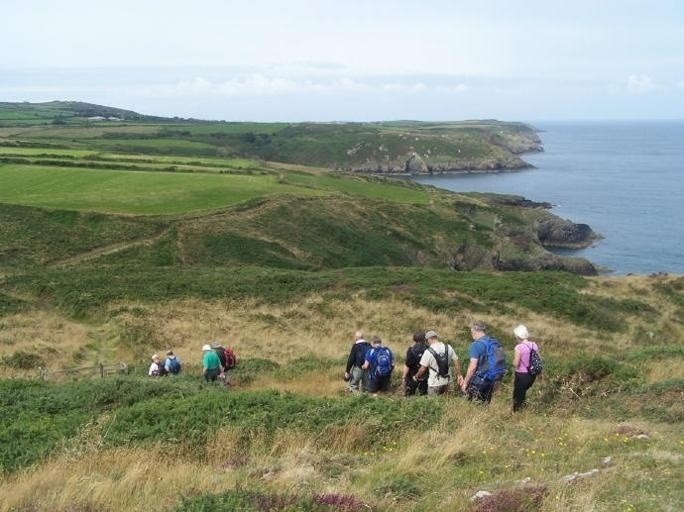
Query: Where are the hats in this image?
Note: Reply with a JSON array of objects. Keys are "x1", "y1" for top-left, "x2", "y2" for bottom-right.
[
  {"x1": 513, "y1": 324, "x2": 529, "y2": 339},
  {"x1": 211, "y1": 341, "x2": 222, "y2": 349},
  {"x1": 424, "y1": 330, "x2": 438, "y2": 339},
  {"x1": 202, "y1": 344, "x2": 212, "y2": 351}
]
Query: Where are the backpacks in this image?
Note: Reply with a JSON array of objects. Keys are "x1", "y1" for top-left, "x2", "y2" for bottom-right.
[
  {"x1": 221, "y1": 347, "x2": 237, "y2": 371},
  {"x1": 411, "y1": 346, "x2": 429, "y2": 381},
  {"x1": 519, "y1": 342, "x2": 543, "y2": 376},
  {"x1": 476, "y1": 336, "x2": 506, "y2": 383},
  {"x1": 152, "y1": 361, "x2": 167, "y2": 377},
  {"x1": 168, "y1": 356, "x2": 181, "y2": 374},
  {"x1": 370, "y1": 346, "x2": 395, "y2": 379},
  {"x1": 426, "y1": 342, "x2": 449, "y2": 378}
]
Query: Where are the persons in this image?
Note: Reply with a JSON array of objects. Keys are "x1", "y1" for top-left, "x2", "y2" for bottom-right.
[
  {"x1": 411, "y1": 330, "x2": 464, "y2": 397},
  {"x1": 512, "y1": 323, "x2": 544, "y2": 412},
  {"x1": 201, "y1": 344, "x2": 226, "y2": 385},
  {"x1": 164, "y1": 350, "x2": 182, "y2": 374},
  {"x1": 211, "y1": 342, "x2": 232, "y2": 380},
  {"x1": 148, "y1": 354, "x2": 167, "y2": 376},
  {"x1": 361, "y1": 335, "x2": 395, "y2": 393},
  {"x1": 458, "y1": 323, "x2": 500, "y2": 408},
  {"x1": 402, "y1": 332, "x2": 432, "y2": 397},
  {"x1": 343, "y1": 330, "x2": 370, "y2": 391}
]
